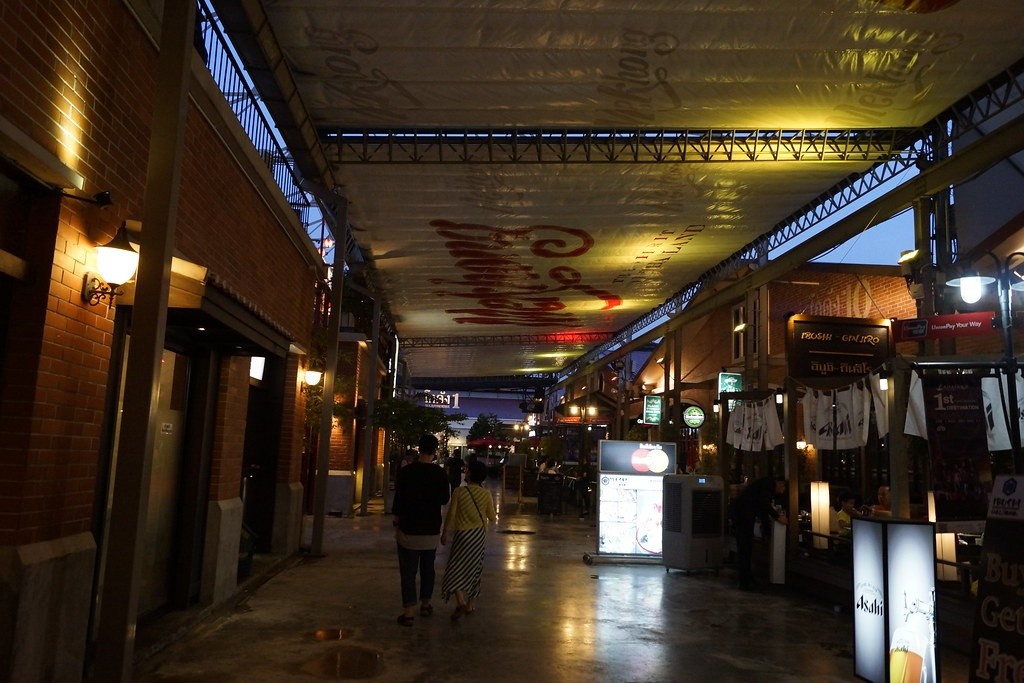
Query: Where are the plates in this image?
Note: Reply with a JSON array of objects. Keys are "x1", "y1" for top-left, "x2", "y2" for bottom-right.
[{"x1": 637, "y1": 528, "x2": 662, "y2": 554}]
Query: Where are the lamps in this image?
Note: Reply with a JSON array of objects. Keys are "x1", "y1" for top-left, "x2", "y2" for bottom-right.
[
  {"x1": 56, "y1": 191, "x2": 115, "y2": 210},
  {"x1": 810, "y1": 480, "x2": 830, "y2": 550},
  {"x1": 81, "y1": 220, "x2": 140, "y2": 310},
  {"x1": 301, "y1": 359, "x2": 322, "y2": 393},
  {"x1": 897, "y1": 248, "x2": 922, "y2": 265},
  {"x1": 733, "y1": 322, "x2": 757, "y2": 332},
  {"x1": 656, "y1": 357, "x2": 665, "y2": 366}
]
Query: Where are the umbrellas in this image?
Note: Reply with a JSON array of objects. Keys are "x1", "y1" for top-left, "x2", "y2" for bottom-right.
[{"x1": 465, "y1": 435, "x2": 508, "y2": 465}]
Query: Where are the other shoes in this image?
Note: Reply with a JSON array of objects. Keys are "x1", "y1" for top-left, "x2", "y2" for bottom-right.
[
  {"x1": 450, "y1": 604, "x2": 466, "y2": 621},
  {"x1": 397, "y1": 613, "x2": 413, "y2": 627},
  {"x1": 465, "y1": 606, "x2": 476, "y2": 616},
  {"x1": 420, "y1": 604, "x2": 433, "y2": 617}
]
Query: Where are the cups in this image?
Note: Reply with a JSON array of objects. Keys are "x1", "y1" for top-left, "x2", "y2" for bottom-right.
[{"x1": 890, "y1": 628, "x2": 927, "y2": 683}]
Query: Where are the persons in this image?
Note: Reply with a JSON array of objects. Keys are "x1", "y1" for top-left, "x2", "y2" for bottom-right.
[
  {"x1": 859, "y1": 484, "x2": 891, "y2": 519},
  {"x1": 731, "y1": 482, "x2": 789, "y2": 590},
  {"x1": 439, "y1": 459, "x2": 495, "y2": 620},
  {"x1": 398, "y1": 449, "x2": 419, "y2": 468},
  {"x1": 445, "y1": 449, "x2": 466, "y2": 497},
  {"x1": 390, "y1": 435, "x2": 449, "y2": 627},
  {"x1": 577, "y1": 454, "x2": 592, "y2": 518},
  {"x1": 836, "y1": 491, "x2": 854, "y2": 536}
]
